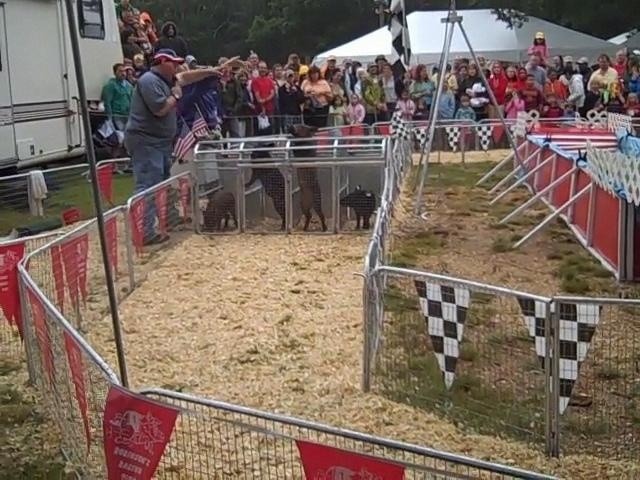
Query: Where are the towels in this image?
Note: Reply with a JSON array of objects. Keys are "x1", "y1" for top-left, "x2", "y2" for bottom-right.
[
  {"x1": 387, "y1": 0, "x2": 410, "y2": 66},
  {"x1": 28, "y1": 170, "x2": 48, "y2": 218}
]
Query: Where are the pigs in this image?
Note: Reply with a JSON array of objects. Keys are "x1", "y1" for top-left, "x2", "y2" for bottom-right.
[
  {"x1": 340, "y1": 184, "x2": 376, "y2": 231},
  {"x1": 243, "y1": 142, "x2": 286, "y2": 230},
  {"x1": 286, "y1": 122, "x2": 328, "y2": 232},
  {"x1": 201, "y1": 189, "x2": 239, "y2": 232}
]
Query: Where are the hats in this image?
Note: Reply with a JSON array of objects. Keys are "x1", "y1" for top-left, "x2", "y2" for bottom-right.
[
  {"x1": 534, "y1": 30, "x2": 545, "y2": 42},
  {"x1": 298, "y1": 64, "x2": 309, "y2": 76},
  {"x1": 325, "y1": 56, "x2": 388, "y2": 72},
  {"x1": 155, "y1": 48, "x2": 185, "y2": 63},
  {"x1": 464, "y1": 82, "x2": 487, "y2": 95},
  {"x1": 565, "y1": 54, "x2": 589, "y2": 65}
]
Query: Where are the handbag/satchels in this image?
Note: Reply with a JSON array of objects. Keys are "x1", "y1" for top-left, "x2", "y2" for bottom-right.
[
  {"x1": 229, "y1": 116, "x2": 247, "y2": 136},
  {"x1": 255, "y1": 115, "x2": 272, "y2": 130}
]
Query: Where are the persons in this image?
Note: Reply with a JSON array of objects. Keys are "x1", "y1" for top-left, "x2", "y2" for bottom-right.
[
  {"x1": 218, "y1": 51, "x2": 386, "y2": 138},
  {"x1": 99, "y1": 2, "x2": 204, "y2": 137},
  {"x1": 378, "y1": 30, "x2": 639, "y2": 136},
  {"x1": 120, "y1": 46, "x2": 231, "y2": 247}
]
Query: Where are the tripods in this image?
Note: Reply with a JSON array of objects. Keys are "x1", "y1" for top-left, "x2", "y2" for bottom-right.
[{"x1": 410, "y1": 24, "x2": 538, "y2": 216}]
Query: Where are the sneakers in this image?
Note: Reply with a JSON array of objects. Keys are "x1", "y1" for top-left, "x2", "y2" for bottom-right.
[
  {"x1": 167, "y1": 217, "x2": 191, "y2": 231},
  {"x1": 143, "y1": 234, "x2": 172, "y2": 246}
]
